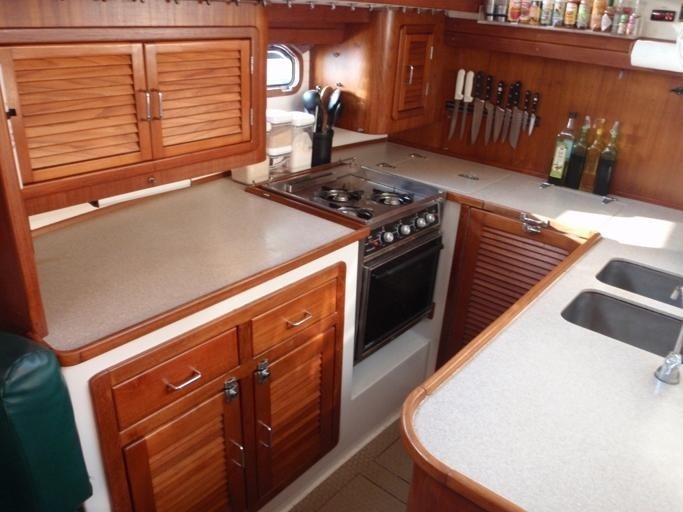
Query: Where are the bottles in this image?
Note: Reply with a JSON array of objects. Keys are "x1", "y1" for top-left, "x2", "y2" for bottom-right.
[
  {"x1": 592, "y1": 120, "x2": 621, "y2": 196},
  {"x1": 548, "y1": 111, "x2": 577, "y2": 187},
  {"x1": 576, "y1": 117, "x2": 606, "y2": 193},
  {"x1": 484, "y1": 0, "x2": 641, "y2": 37},
  {"x1": 561, "y1": 115, "x2": 592, "y2": 190}
]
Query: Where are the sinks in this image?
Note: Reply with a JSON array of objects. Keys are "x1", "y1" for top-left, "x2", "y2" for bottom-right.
[
  {"x1": 559, "y1": 286, "x2": 683, "y2": 369},
  {"x1": 594, "y1": 256, "x2": 683, "y2": 316}
]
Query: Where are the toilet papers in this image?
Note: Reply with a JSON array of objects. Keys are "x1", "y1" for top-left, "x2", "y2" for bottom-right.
[{"x1": 629, "y1": 38, "x2": 683, "y2": 74}]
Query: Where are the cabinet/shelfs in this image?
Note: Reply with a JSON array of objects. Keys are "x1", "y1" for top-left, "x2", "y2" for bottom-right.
[
  {"x1": 89, "y1": 261, "x2": 347, "y2": 510},
  {"x1": 0, "y1": 23, "x2": 259, "y2": 198},
  {"x1": 310, "y1": 9, "x2": 446, "y2": 140},
  {"x1": 435, "y1": 207, "x2": 582, "y2": 368}
]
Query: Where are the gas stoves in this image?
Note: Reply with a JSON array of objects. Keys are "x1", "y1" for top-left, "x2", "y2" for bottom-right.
[{"x1": 290, "y1": 168, "x2": 447, "y2": 264}]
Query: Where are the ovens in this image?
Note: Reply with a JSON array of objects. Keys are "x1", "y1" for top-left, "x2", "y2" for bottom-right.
[{"x1": 352, "y1": 230, "x2": 444, "y2": 367}]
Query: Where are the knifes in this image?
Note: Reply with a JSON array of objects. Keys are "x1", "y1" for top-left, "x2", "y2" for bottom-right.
[{"x1": 447, "y1": 69, "x2": 540, "y2": 149}]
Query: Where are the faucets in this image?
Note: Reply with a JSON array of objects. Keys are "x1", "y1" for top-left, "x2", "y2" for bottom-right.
[{"x1": 668, "y1": 285, "x2": 683, "y2": 302}]
